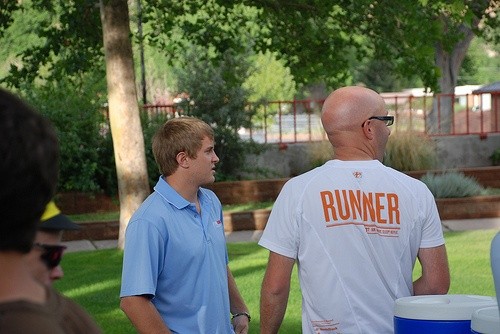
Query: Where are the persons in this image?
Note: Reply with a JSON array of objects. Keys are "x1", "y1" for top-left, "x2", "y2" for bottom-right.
[
  {"x1": 119, "y1": 117, "x2": 250, "y2": 334},
  {"x1": 0, "y1": 88, "x2": 103, "y2": 334},
  {"x1": 32, "y1": 205, "x2": 76, "y2": 289},
  {"x1": 259, "y1": 86, "x2": 451, "y2": 334}
]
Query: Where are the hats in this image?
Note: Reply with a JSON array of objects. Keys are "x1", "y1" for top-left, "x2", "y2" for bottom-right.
[{"x1": 35, "y1": 201, "x2": 87, "y2": 231}]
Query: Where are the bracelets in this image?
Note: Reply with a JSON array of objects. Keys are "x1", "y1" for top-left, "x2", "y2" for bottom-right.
[{"x1": 229, "y1": 311, "x2": 252, "y2": 319}]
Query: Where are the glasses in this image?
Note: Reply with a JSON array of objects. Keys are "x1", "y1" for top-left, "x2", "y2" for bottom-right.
[
  {"x1": 361, "y1": 115, "x2": 395, "y2": 128},
  {"x1": 29, "y1": 241, "x2": 66, "y2": 270}
]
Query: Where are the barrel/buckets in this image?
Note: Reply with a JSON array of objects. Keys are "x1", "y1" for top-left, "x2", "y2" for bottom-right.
[
  {"x1": 394, "y1": 295, "x2": 498, "y2": 334},
  {"x1": 470, "y1": 307, "x2": 500, "y2": 334}
]
